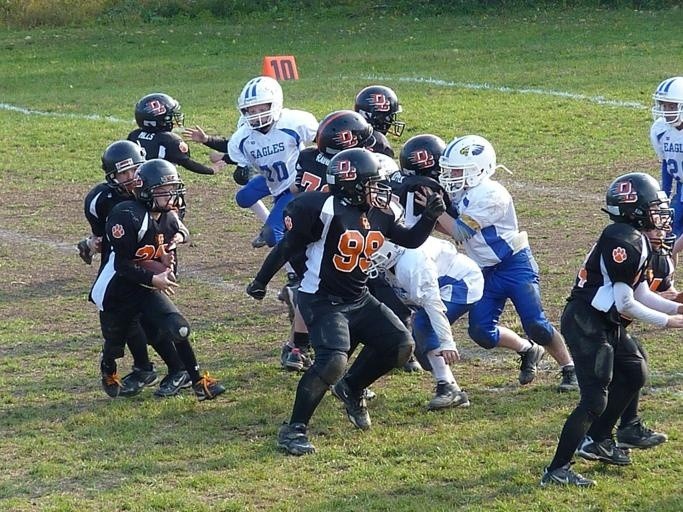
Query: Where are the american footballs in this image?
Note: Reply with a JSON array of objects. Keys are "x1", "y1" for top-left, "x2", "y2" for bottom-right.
[{"x1": 133, "y1": 260, "x2": 176, "y2": 290}]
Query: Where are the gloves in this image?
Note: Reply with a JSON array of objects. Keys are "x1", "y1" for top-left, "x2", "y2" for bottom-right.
[
  {"x1": 426, "y1": 194, "x2": 445, "y2": 219},
  {"x1": 246, "y1": 279, "x2": 265, "y2": 299}
]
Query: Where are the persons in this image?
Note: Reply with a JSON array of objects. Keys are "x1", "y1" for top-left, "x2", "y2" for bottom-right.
[
  {"x1": 79, "y1": 92, "x2": 229, "y2": 404},
  {"x1": 540, "y1": 172, "x2": 683, "y2": 489},
  {"x1": 649, "y1": 76, "x2": 683, "y2": 282},
  {"x1": 182, "y1": 76, "x2": 578, "y2": 455}
]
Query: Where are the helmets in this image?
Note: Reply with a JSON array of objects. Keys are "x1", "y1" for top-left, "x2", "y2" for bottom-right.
[
  {"x1": 236, "y1": 75, "x2": 495, "y2": 210},
  {"x1": 98, "y1": 92, "x2": 189, "y2": 214},
  {"x1": 599, "y1": 75, "x2": 683, "y2": 258}
]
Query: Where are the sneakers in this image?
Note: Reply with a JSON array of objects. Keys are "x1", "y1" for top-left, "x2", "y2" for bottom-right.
[
  {"x1": 331, "y1": 377, "x2": 372, "y2": 433},
  {"x1": 280, "y1": 342, "x2": 315, "y2": 371},
  {"x1": 277, "y1": 421, "x2": 316, "y2": 456},
  {"x1": 516, "y1": 339, "x2": 546, "y2": 385},
  {"x1": 541, "y1": 460, "x2": 598, "y2": 488},
  {"x1": 363, "y1": 387, "x2": 377, "y2": 400},
  {"x1": 574, "y1": 434, "x2": 632, "y2": 465},
  {"x1": 98, "y1": 349, "x2": 226, "y2": 402},
  {"x1": 613, "y1": 415, "x2": 668, "y2": 449},
  {"x1": 405, "y1": 354, "x2": 422, "y2": 372},
  {"x1": 252, "y1": 232, "x2": 265, "y2": 248},
  {"x1": 74, "y1": 236, "x2": 93, "y2": 264},
  {"x1": 429, "y1": 380, "x2": 470, "y2": 412},
  {"x1": 555, "y1": 365, "x2": 580, "y2": 393}
]
